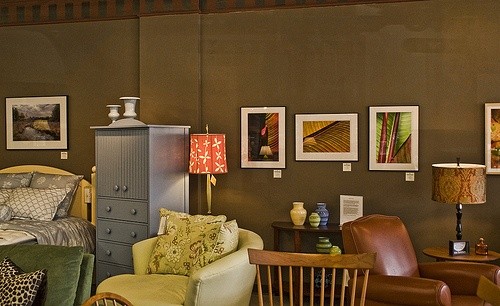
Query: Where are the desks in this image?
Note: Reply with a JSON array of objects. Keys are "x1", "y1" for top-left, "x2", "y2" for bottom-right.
[
  {"x1": 269, "y1": 221, "x2": 350, "y2": 306},
  {"x1": 421, "y1": 247, "x2": 500, "y2": 262}
]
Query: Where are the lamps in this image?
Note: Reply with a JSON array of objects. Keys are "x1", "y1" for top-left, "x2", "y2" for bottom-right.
[
  {"x1": 432, "y1": 157, "x2": 488, "y2": 240},
  {"x1": 189, "y1": 125, "x2": 229, "y2": 214}
]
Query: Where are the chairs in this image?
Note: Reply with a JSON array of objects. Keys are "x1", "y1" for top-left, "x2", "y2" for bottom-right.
[
  {"x1": 0, "y1": 245, "x2": 95, "y2": 306},
  {"x1": 81, "y1": 292, "x2": 134, "y2": 306},
  {"x1": 247, "y1": 247, "x2": 379, "y2": 306},
  {"x1": 476, "y1": 274, "x2": 500, "y2": 306}
]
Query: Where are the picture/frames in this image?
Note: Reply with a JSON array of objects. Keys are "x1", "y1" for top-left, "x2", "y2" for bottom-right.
[
  {"x1": 240, "y1": 107, "x2": 287, "y2": 170},
  {"x1": 5, "y1": 96, "x2": 68, "y2": 150},
  {"x1": 368, "y1": 104, "x2": 420, "y2": 173},
  {"x1": 485, "y1": 103, "x2": 500, "y2": 174},
  {"x1": 294, "y1": 112, "x2": 360, "y2": 162}
]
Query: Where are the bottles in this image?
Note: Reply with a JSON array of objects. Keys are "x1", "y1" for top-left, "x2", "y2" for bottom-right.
[
  {"x1": 290, "y1": 202, "x2": 307, "y2": 226},
  {"x1": 314, "y1": 268, "x2": 328, "y2": 289},
  {"x1": 329, "y1": 246, "x2": 342, "y2": 255},
  {"x1": 315, "y1": 203, "x2": 329, "y2": 226},
  {"x1": 315, "y1": 237, "x2": 332, "y2": 254},
  {"x1": 309, "y1": 212, "x2": 321, "y2": 228}
]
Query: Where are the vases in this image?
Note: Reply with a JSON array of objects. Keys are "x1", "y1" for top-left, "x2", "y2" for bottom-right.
[
  {"x1": 308, "y1": 201, "x2": 329, "y2": 226},
  {"x1": 289, "y1": 200, "x2": 307, "y2": 226},
  {"x1": 315, "y1": 270, "x2": 332, "y2": 288},
  {"x1": 106, "y1": 104, "x2": 122, "y2": 124},
  {"x1": 119, "y1": 95, "x2": 141, "y2": 119},
  {"x1": 316, "y1": 237, "x2": 341, "y2": 253}
]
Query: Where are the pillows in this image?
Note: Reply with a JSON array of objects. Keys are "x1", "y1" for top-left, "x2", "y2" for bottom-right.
[
  {"x1": 29, "y1": 171, "x2": 84, "y2": 218},
  {"x1": 0, "y1": 172, "x2": 33, "y2": 189},
  {"x1": 147, "y1": 207, "x2": 240, "y2": 276},
  {"x1": 5, "y1": 188, "x2": 72, "y2": 222},
  {"x1": 0, "y1": 189, "x2": 13, "y2": 205},
  {"x1": 0, "y1": 257, "x2": 48, "y2": 306}
]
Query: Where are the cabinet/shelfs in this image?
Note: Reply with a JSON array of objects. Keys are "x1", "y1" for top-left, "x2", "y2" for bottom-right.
[{"x1": 91, "y1": 124, "x2": 192, "y2": 284}]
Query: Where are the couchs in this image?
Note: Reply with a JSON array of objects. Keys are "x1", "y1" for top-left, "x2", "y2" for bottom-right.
[
  {"x1": 96, "y1": 227, "x2": 265, "y2": 306},
  {"x1": 341, "y1": 214, "x2": 500, "y2": 306}
]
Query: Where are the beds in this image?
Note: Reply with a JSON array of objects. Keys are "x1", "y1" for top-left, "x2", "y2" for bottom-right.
[{"x1": 0, "y1": 165, "x2": 96, "y2": 254}]
